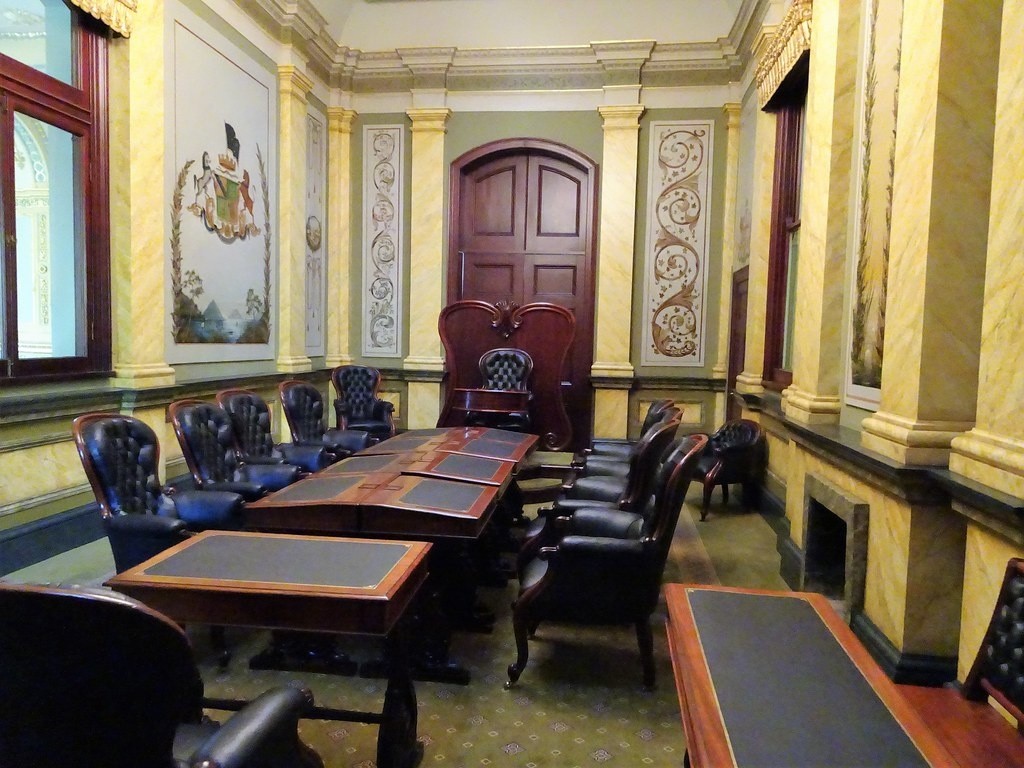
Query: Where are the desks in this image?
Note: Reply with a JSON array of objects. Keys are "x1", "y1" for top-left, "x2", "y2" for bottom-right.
[
  {"x1": 665, "y1": 578, "x2": 956, "y2": 768},
  {"x1": 243, "y1": 427, "x2": 540, "y2": 687},
  {"x1": 103, "y1": 529, "x2": 470, "y2": 767}
]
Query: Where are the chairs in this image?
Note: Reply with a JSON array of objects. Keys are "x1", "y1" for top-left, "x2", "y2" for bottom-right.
[
  {"x1": 896, "y1": 557, "x2": 1024, "y2": 768},
  {"x1": 0, "y1": 584, "x2": 321, "y2": 768},
  {"x1": 71, "y1": 361, "x2": 396, "y2": 574},
  {"x1": 464, "y1": 348, "x2": 761, "y2": 693}
]
